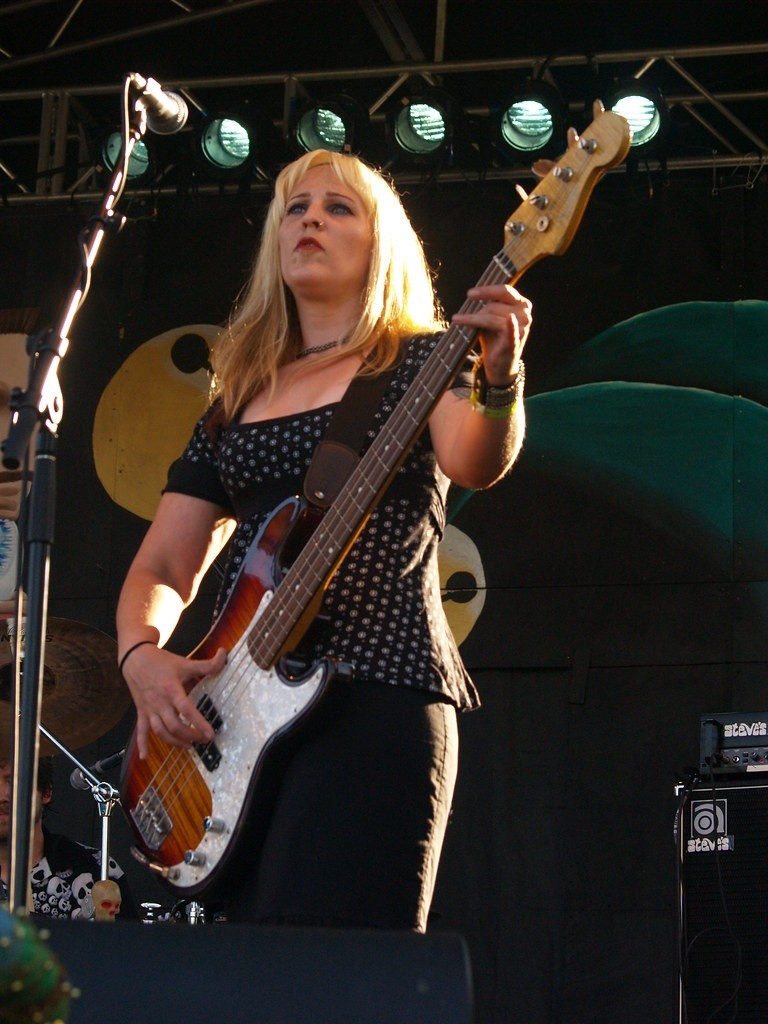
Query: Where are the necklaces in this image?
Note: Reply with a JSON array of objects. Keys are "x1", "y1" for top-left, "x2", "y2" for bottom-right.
[{"x1": 295, "y1": 333, "x2": 349, "y2": 358}]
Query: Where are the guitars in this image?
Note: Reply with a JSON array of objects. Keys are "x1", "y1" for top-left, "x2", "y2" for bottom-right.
[{"x1": 119, "y1": 95, "x2": 634, "y2": 891}]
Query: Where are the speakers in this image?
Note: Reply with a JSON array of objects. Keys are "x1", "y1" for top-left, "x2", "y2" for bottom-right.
[
  {"x1": 21, "y1": 915, "x2": 479, "y2": 1024},
  {"x1": 673, "y1": 775, "x2": 768, "y2": 1024}
]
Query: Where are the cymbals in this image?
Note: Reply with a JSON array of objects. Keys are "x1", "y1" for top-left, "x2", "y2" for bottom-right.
[{"x1": 0, "y1": 613, "x2": 128, "y2": 762}]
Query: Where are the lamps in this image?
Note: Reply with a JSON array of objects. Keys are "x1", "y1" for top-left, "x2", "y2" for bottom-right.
[
  {"x1": 382, "y1": 86, "x2": 473, "y2": 158},
  {"x1": 584, "y1": 74, "x2": 670, "y2": 161},
  {"x1": 492, "y1": 78, "x2": 570, "y2": 167},
  {"x1": 289, "y1": 87, "x2": 383, "y2": 155},
  {"x1": 98, "y1": 125, "x2": 166, "y2": 184},
  {"x1": 200, "y1": 100, "x2": 274, "y2": 173}
]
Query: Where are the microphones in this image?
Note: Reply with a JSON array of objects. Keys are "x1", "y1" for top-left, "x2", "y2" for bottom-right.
[
  {"x1": 123, "y1": 72, "x2": 189, "y2": 134},
  {"x1": 70, "y1": 749, "x2": 125, "y2": 790}
]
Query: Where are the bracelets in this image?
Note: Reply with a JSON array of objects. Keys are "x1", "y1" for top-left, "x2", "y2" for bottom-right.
[
  {"x1": 119, "y1": 640, "x2": 159, "y2": 673},
  {"x1": 471, "y1": 351, "x2": 525, "y2": 418}
]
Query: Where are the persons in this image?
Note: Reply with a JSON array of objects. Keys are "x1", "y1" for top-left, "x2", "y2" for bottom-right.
[
  {"x1": 110, "y1": 149, "x2": 532, "y2": 935},
  {"x1": 0, "y1": 755, "x2": 128, "y2": 920}
]
